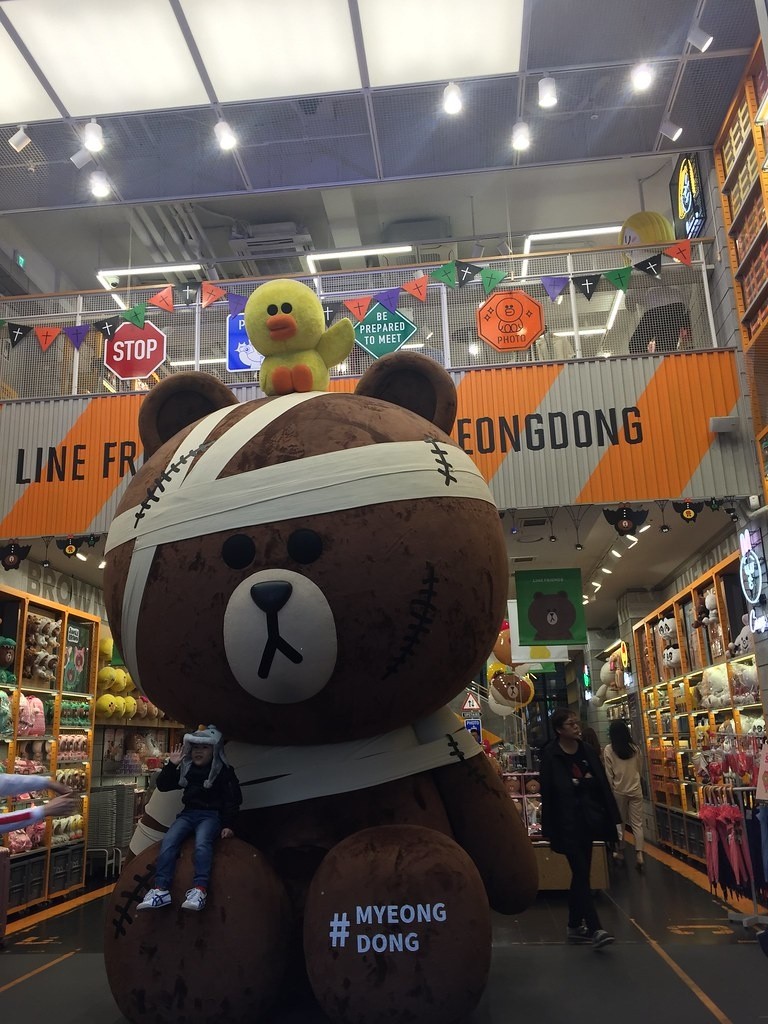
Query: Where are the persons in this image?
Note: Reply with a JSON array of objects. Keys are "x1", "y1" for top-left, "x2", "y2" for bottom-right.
[
  {"x1": 135, "y1": 725, "x2": 243, "y2": 910},
  {"x1": 603, "y1": 719, "x2": 646, "y2": 874},
  {"x1": 539, "y1": 708, "x2": 614, "y2": 948}
]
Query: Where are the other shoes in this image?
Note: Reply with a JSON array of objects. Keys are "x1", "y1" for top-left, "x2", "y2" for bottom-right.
[
  {"x1": 613, "y1": 853, "x2": 626, "y2": 859},
  {"x1": 635, "y1": 860, "x2": 645, "y2": 871}
]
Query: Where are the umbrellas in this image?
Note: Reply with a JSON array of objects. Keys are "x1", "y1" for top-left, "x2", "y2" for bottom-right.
[{"x1": 700, "y1": 784, "x2": 754, "y2": 904}]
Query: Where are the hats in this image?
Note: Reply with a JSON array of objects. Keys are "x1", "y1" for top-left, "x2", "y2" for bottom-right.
[{"x1": 179, "y1": 724, "x2": 230, "y2": 787}]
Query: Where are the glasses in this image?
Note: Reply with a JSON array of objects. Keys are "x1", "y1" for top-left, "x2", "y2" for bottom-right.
[{"x1": 563, "y1": 720, "x2": 580, "y2": 727}]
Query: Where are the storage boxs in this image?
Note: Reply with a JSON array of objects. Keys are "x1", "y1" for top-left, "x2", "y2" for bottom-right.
[{"x1": 503, "y1": 773, "x2": 542, "y2": 835}]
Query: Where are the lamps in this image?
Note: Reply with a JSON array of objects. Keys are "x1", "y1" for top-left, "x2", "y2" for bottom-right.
[
  {"x1": 660, "y1": 116, "x2": 682, "y2": 140},
  {"x1": 444, "y1": 82, "x2": 461, "y2": 113},
  {"x1": 212, "y1": 116, "x2": 237, "y2": 151},
  {"x1": 85, "y1": 117, "x2": 103, "y2": 151},
  {"x1": 8, "y1": 125, "x2": 31, "y2": 153},
  {"x1": 686, "y1": 25, "x2": 713, "y2": 54},
  {"x1": 70, "y1": 149, "x2": 93, "y2": 169},
  {"x1": 513, "y1": 119, "x2": 529, "y2": 151},
  {"x1": 91, "y1": 164, "x2": 110, "y2": 197},
  {"x1": 537, "y1": 70, "x2": 558, "y2": 108}
]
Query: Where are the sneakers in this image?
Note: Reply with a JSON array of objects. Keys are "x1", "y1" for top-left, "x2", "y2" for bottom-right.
[
  {"x1": 136, "y1": 889, "x2": 172, "y2": 910},
  {"x1": 591, "y1": 930, "x2": 615, "y2": 953},
  {"x1": 181, "y1": 889, "x2": 208, "y2": 911},
  {"x1": 566, "y1": 926, "x2": 592, "y2": 940}
]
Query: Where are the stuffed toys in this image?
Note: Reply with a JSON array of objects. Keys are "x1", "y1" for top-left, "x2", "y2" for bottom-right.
[
  {"x1": 505, "y1": 773, "x2": 540, "y2": 819},
  {"x1": 0, "y1": 613, "x2": 172, "y2": 855},
  {"x1": 588, "y1": 586, "x2": 765, "y2": 749},
  {"x1": 244, "y1": 279, "x2": 355, "y2": 397},
  {"x1": 617, "y1": 211, "x2": 684, "y2": 353},
  {"x1": 104, "y1": 351, "x2": 540, "y2": 1024}
]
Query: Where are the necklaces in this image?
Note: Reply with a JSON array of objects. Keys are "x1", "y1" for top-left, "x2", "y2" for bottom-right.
[{"x1": 559, "y1": 742, "x2": 576, "y2": 754}]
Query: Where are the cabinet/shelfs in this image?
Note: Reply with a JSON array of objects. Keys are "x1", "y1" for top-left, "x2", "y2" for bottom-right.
[
  {"x1": 631, "y1": 547, "x2": 767, "y2": 865},
  {"x1": 0, "y1": 583, "x2": 101, "y2": 916},
  {"x1": 531, "y1": 840, "x2": 608, "y2": 901}
]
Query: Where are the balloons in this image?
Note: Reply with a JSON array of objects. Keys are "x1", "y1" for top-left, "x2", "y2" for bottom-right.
[{"x1": 487, "y1": 620, "x2": 534, "y2": 716}]
